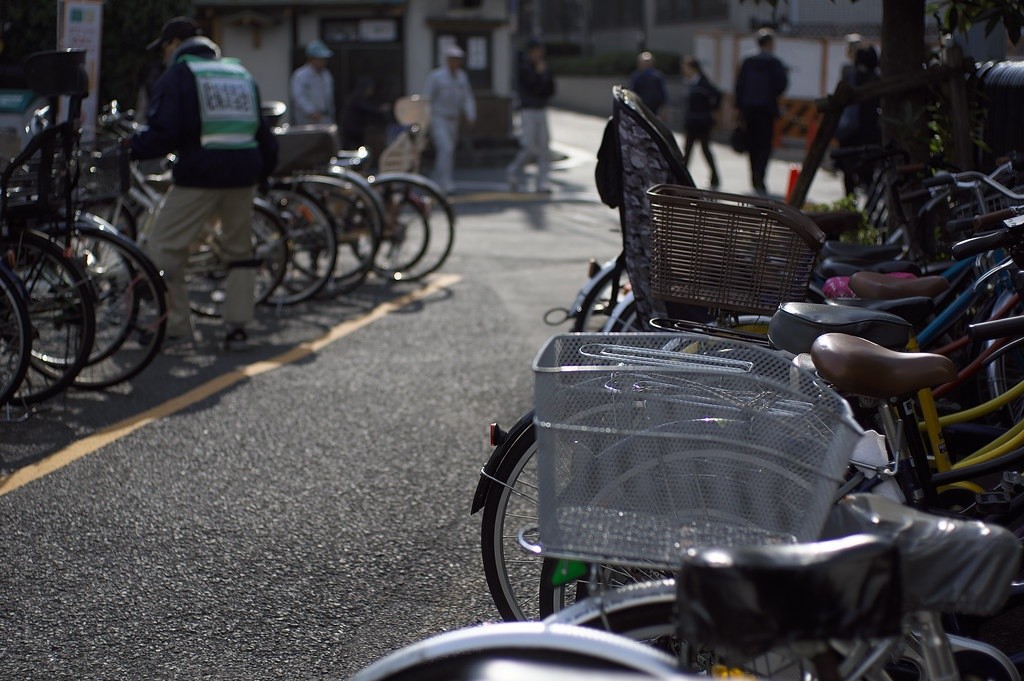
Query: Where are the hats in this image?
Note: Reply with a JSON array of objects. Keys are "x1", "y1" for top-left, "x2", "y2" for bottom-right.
[
  {"x1": 446, "y1": 47, "x2": 466, "y2": 59},
  {"x1": 304, "y1": 39, "x2": 334, "y2": 58},
  {"x1": 145, "y1": 16, "x2": 201, "y2": 51}
]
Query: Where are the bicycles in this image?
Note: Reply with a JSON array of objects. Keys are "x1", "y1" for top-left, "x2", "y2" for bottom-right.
[{"x1": 0, "y1": 86, "x2": 1023, "y2": 681}]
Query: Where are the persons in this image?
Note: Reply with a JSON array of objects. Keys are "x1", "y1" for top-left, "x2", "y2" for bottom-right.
[
  {"x1": 418, "y1": 46, "x2": 485, "y2": 195},
  {"x1": 109, "y1": 17, "x2": 270, "y2": 353},
  {"x1": 731, "y1": 29, "x2": 788, "y2": 193},
  {"x1": 842, "y1": 36, "x2": 879, "y2": 213},
  {"x1": 508, "y1": 37, "x2": 555, "y2": 193},
  {"x1": 628, "y1": 51, "x2": 665, "y2": 117},
  {"x1": 288, "y1": 39, "x2": 338, "y2": 153},
  {"x1": 676, "y1": 59, "x2": 722, "y2": 185}
]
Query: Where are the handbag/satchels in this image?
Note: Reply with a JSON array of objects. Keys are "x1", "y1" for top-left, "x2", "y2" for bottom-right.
[{"x1": 731, "y1": 123, "x2": 748, "y2": 153}]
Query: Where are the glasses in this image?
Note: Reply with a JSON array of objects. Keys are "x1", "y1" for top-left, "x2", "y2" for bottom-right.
[{"x1": 156, "y1": 40, "x2": 172, "y2": 56}]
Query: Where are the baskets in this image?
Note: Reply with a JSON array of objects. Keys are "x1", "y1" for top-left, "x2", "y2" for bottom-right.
[
  {"x1": 533, "y1": 331, "x2": 862, "y2": 572},
  {"x1": 1, "y1": 136, "x2": 120, "y2": 206},
  {"x1": 647, "y1": 182, "x2": 826, "y2": 313}
]
